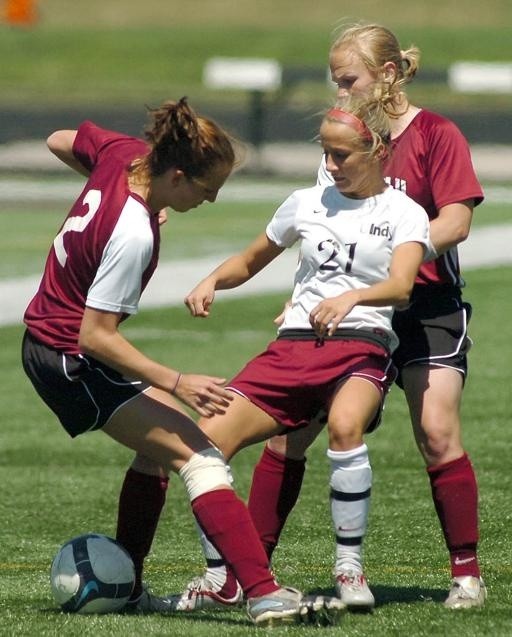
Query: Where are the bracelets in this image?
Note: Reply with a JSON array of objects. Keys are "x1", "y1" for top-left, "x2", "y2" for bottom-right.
[
  {"x1": 422, "y1": 241, "x2": 441, "y2": 266},
  {"x1": 170, "y1": 370, "x2": 184, "y2": 396}
]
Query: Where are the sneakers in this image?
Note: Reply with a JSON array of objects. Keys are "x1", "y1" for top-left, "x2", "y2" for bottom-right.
[
  {"x1": 443, "y1": 575, "x2": 488, "y2": 611},
  {"x1": 246, "y1": 584, "x2": 348, "y2": 628},
  {"x1": 330, "y1": 569, "x2": 376, "y2": 609},
  {"x1": 119, "y1": 576, "x2": 244, "y2": 613}
]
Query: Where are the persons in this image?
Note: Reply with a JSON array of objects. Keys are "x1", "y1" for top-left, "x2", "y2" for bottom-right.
[
  {"x1": 21, "y1": 94, "x2": 348, "y2": 625},
  {"x1": 166, "y1": 79, "x2": 429, "y2": 615},
  {"x1": 247, "y1": 23, "x2": 489, "y2": 612}
]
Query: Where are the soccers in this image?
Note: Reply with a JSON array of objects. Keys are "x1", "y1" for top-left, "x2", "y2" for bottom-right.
[{"x1": 52, "y1": 534, "x2": 135, "y2": 614}]
